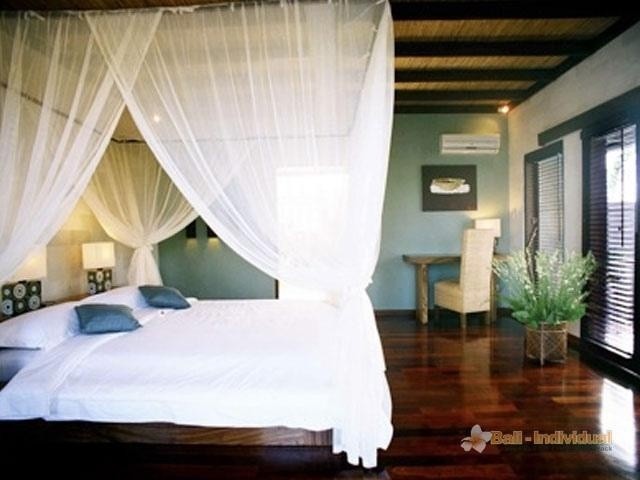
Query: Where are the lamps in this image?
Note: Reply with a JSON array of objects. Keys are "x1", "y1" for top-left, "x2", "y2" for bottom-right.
[
  {"x1": 81, "y1": 240, "x2": 117, "y2": 295},
  {"x1": 474, "y1": 217, "x2": 501, "y2": 255}
]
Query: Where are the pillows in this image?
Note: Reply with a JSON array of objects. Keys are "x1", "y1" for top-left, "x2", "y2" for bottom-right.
[{"x1": 0, "y1": 285, "x2": 191, "y2": 356}]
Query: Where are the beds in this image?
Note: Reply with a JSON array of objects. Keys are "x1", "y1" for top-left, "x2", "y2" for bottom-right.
[{"x1": 0, "y1": 284, "x2": 396, "y2": 469}]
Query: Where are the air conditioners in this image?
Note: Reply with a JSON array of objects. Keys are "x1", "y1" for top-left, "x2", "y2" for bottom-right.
[{"x1": 439, "y1": 134, "x2": 501, "y2": 156}]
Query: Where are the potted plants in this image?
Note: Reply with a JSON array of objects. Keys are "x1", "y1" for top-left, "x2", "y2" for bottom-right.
[{"x1": 485, "y1": 227, "x2": 605, "y2": 366}]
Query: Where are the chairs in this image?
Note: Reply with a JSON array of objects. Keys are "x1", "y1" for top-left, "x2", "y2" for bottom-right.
[{"x1": 433, "y1": 228, "x2": 495, "y2": 330}]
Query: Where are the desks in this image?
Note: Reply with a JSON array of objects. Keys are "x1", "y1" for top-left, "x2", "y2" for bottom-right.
[{"x1": 402, "y1": 254, "x2": 506, "y2": 323}]
